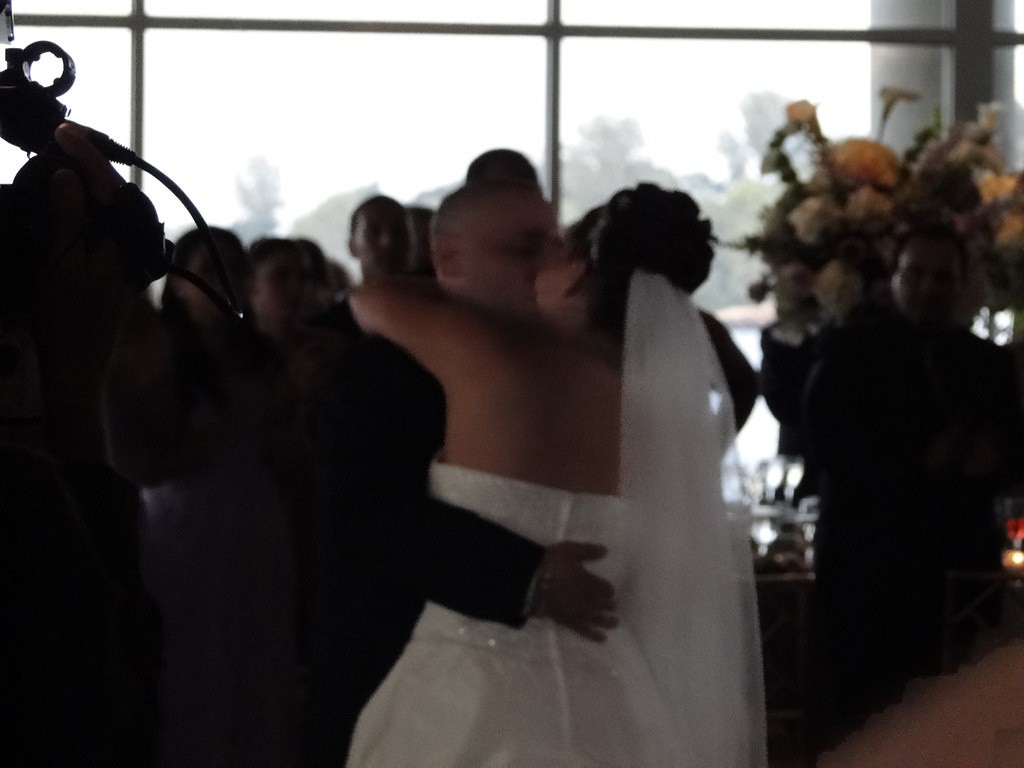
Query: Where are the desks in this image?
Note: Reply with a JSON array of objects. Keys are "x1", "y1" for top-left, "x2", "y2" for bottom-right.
[{"x1": 756, "y1": 566, "x2": 1023, "y2": 768}]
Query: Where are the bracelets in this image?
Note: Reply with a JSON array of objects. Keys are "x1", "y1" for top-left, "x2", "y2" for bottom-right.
[{"x1": 537, "y1": 547, "x2": 554, "y2": 619}]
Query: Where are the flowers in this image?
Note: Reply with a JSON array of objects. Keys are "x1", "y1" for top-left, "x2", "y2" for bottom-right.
[{"x1": 739, "y1": 87, "x2": 1024, "y2": 346}]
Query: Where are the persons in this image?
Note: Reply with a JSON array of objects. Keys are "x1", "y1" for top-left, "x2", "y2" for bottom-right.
[
  {"x1": 763, "y1": 224, "x2": 1024, "y2": 768},
  {"x1": 0, "y1": 123, "x2": 757, "y2": 768}
]
